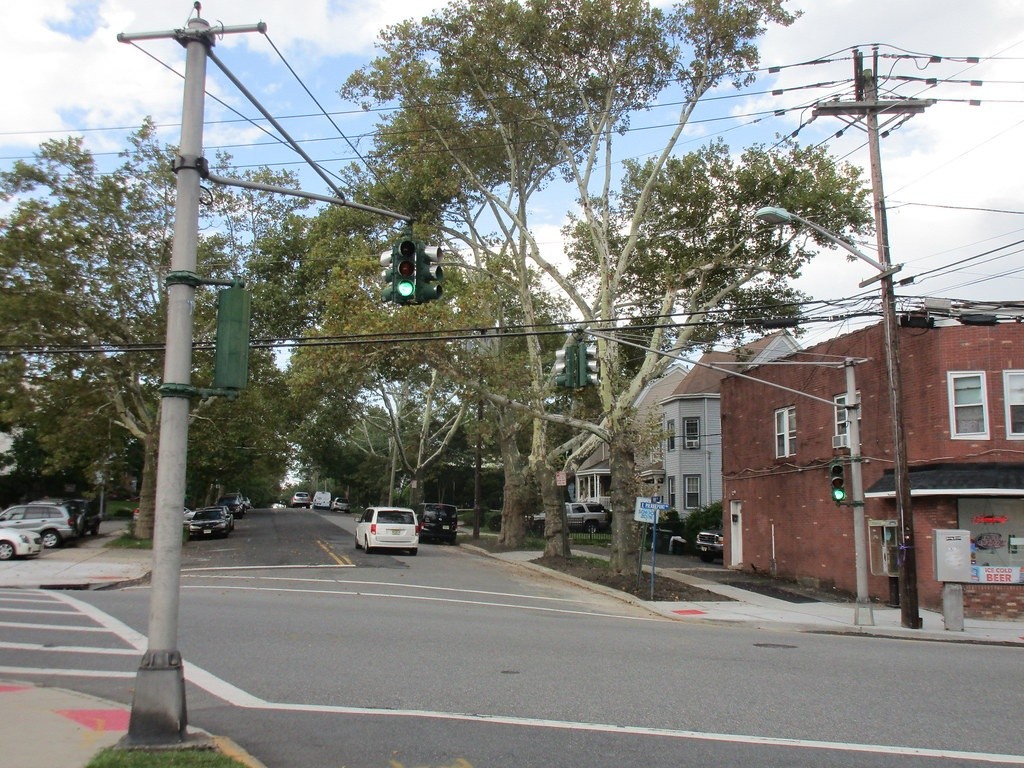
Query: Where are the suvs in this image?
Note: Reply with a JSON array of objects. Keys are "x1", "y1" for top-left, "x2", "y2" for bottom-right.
[{"x1": 0, "y1": 499, "x2": 88, "y2": 549}]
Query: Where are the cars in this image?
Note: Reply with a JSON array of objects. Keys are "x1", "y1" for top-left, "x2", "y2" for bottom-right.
[
  {"x1": 695, "y1": 524, "x2": 724, "y2": 562},
  {"x1": 551, "y1": 503, "x2": 613, "y2": 526},
  {"x1": 182, "y1": 489, "x2": 248, "y2": 540},
  {"x1": 291, "y1": 492, "x2": 311, "y2": 509},
  {"x1": 0, "y1": 526, "x2": 42, "y2": 561},
  {"x1": 331, "y1": 497, "x2": 350, "y2": 512}
]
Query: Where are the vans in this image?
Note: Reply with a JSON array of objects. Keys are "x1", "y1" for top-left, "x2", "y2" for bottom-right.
[
  {"x1": 312, "y1": 491, "x2": 332, "y2": 510},
  {"x1": 416, "y1": 503, "x2": 458, "y2": 545},
  {"x1": 354, "y1": 507, "x2": 419, "y2": 555}
]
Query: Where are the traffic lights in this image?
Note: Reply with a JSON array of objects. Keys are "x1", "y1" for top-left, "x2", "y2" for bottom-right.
[
  {"x1": 831, "y1": 462, "x2": 846, "y2": 501},
  {"x1": 577, "y1": 341, "x2": 600, "y2": 387},
  {"x1": 379, "y1": 235, "x2": 446, "y2": 306},
  {"x1": 556, "y1": 345, "x2": 576, "y2": 389}
]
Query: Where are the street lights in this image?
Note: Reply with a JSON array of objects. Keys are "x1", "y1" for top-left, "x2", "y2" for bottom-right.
[{"x1": 759, "y1": 206, "x2": 926, "y2": 629}]
